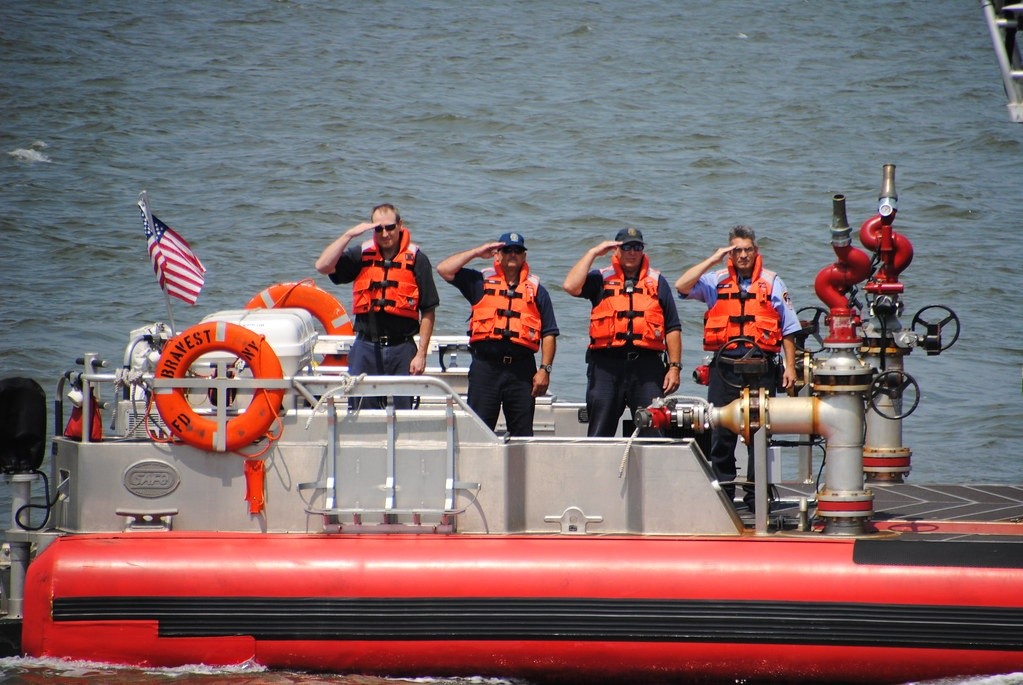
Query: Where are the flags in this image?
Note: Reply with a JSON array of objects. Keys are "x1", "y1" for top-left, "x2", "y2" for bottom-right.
[{"x1": 137, "y1": 201, "x2": 206, "y2": 306}]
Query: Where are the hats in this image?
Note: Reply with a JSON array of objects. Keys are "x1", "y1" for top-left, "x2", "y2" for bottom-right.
[
  {"x1": 614, "y1": 227, "x2": 644, "y2": 244},
  {"x1": 497, "y1": 232, "x2": 527, "y2": 251}
]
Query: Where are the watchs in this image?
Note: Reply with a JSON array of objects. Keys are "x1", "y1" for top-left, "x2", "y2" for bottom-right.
[
  {"x1": 670, "y1": 361, "x2": 682, "y2": 370},
  {"x1": 540, "y1": 364, "x2": 552, "y2": 373}
]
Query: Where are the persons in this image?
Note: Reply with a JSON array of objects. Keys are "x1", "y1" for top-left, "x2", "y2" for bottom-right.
[
  {"x1": 674, "y1": 226, "x2": 803, "y2": 513},
  {"x1": 315, "y1": 203, "x2": 440, "y2": 410},
  {"x1": 563, "y1": 229, "x2": 684, "y2": 437},
  {"x1": 436, "y1": 232, "x2": 559, "y2": 437}
]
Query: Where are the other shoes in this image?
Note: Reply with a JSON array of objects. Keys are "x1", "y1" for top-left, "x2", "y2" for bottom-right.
[{"x1": 748, "y1": 501, "x2": 774, "y2": 514}]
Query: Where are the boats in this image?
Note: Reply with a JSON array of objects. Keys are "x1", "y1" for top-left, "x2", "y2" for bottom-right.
[{"x1": 0, "y1": 280, "x2": 1023, "y2": 683}]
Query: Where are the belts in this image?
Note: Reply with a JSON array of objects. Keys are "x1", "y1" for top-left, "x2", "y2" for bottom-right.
[
  {"x1": 358, "y1": 331, "x2": 413, "y2": 346},
  {"x1": 476, "y1": 351, "x2": 534, "y2": 365},
  {"x1": 591, "y1": 350, "x2": 640, "y2": 361}
]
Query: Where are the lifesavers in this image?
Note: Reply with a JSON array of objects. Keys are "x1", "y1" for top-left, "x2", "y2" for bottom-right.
[
  {"x1": 243, "y1": 282, "x2": 356, "y2": 375},
  {"x1": 153, "y1": 319, "x2": 286, "y2": 453}
]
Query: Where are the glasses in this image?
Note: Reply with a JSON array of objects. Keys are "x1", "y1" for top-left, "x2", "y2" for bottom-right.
[
  {"x1": 620, "y1": 243, "x2": 644, "y2": 251},
  {"x1": 502, "y1": 247, "x2": 524, "y2": 253},
  {"x1": 375, "y1": 224, "x2": 397, "y2": 232}
]
